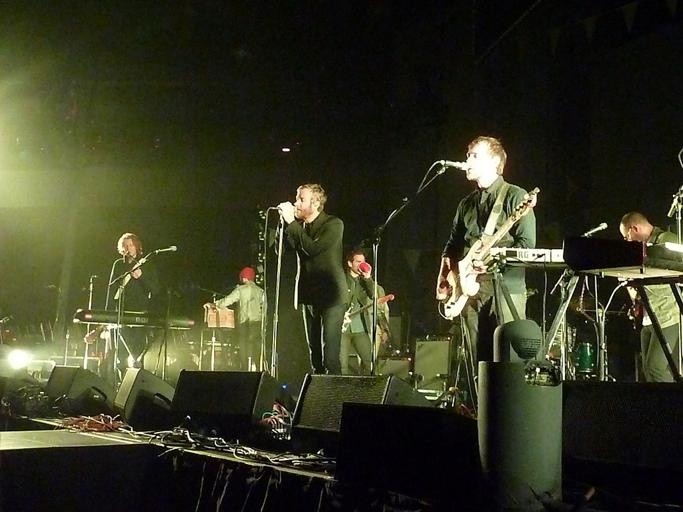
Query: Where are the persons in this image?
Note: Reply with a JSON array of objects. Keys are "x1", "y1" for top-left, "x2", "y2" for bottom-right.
[
  {"x1": 434, "y1": 136, "x2": 538, "y2": 397},
  {"x1": 617, "y1": 210, "x2": 682, "y2": 382},
  {"x1": 104, "y1": 233, "x2": 161, "y2": 378},
  {"x1": 357, "y1": 283, "x2": 392, "y2": 374},
  {"x1": 201, "y1": 267, "x2": 267, "y2": 371},
  {"x1": 338, "y1": 251, "x2": 373, "y2": 375},
  {"x1": 277, "y1": 185, "x2": 348, "y2": 375}
]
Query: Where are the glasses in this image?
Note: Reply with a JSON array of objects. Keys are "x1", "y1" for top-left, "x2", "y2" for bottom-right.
[{"x1": 622, "y1": 228, "x2": 632, "y2": 242}]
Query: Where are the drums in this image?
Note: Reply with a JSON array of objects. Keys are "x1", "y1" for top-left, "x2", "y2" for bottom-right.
[{"x1": 575, "y1": 343, "x2": 594, "y2": 372}]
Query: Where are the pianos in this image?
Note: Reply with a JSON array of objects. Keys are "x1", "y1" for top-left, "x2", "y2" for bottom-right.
[{"x1": 73, "y1": 308, "x2": 194, "y2": 329}]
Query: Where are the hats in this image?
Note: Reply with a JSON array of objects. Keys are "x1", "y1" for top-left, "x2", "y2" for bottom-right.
[{"x1": 240, "y1": 267, "x2": 255, "y2": 281}]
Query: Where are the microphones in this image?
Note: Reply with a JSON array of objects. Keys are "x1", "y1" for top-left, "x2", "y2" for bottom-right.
[
  {"x1": 274, "y1": 206, "x2": 297, "y2": 214},
  {"x1": 441, "y1": 160, "x2": 470, "y2": 171},
  {"x1": 584, "y1": 221, "x2": 608, "y2": 238},
  {"x1": 156, "y1": 246, "x2": 177, "y2": 253}
]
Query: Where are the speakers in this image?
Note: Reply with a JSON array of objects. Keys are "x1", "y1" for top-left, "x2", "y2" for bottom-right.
[
  {"x1": 114, "y1": 366, "x2": 176, "y2": 422},
  {"x1": 169, "y1": 370, "x2": 295, "y2": 422},
  {"x1": 44, "y1": 364, "x2": 99, "y2": 399},
  {"x1": 291, "y1": 372, "x2": 434, "y2": 452}
]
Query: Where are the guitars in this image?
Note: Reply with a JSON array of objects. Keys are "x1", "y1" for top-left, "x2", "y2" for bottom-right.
[
  {"x1": 84, "y1": 326, "x2": 103, "y2": 345},
  {"x1": 443, "y1": 187, "x2": 540, "y2": 320},
  {"x1": 342, "y1": 294, "x2": 395, "y2": 333}
]
